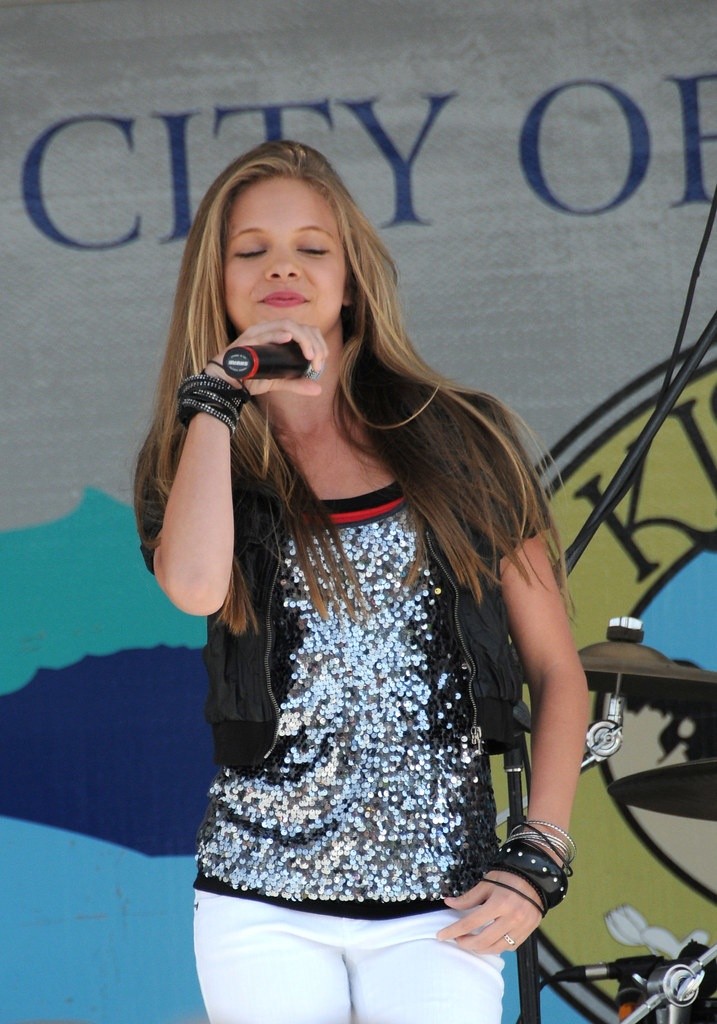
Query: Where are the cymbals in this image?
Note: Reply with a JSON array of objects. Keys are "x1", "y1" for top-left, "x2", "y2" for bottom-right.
[
  {"x1": 605, "y1": 756, "x2": 717, "y2": 822},
  {"x1": 577, "y1": 640, "x2": 717, "y2": 701}
]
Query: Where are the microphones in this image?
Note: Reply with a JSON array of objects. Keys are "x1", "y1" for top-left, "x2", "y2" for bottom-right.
[
  {"x1": 221, "y1": 340, "x2": 327, "y2": 381},
  {"x1": 555, "y1": 955, "x2": 665, "y2": 984}
]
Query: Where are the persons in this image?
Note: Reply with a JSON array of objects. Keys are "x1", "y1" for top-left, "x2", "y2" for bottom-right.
[{"x1": 134, "y1": 138, "x2": 588, "y2": 1024}]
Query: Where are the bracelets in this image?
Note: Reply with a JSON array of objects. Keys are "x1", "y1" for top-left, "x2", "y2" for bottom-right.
[
  {"x1": 480, "y1": 820, "x2": 577, "y2": 919},
  {"x1": 176, "y1": 360, "x2": 250, "y2": 439}
]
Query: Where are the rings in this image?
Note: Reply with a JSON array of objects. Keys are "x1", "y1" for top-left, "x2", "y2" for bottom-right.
[{"x1": 504, "y1": 934, "x2": 515, "y2": 945}]
